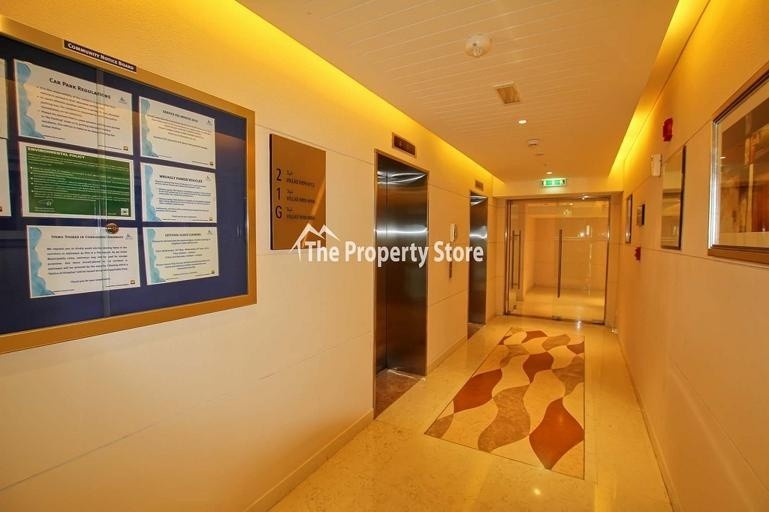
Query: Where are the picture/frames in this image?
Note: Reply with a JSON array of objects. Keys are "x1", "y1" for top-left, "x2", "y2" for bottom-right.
[{"x1": 705, "y1": 60, "x2": 768, "y2": 265}]
